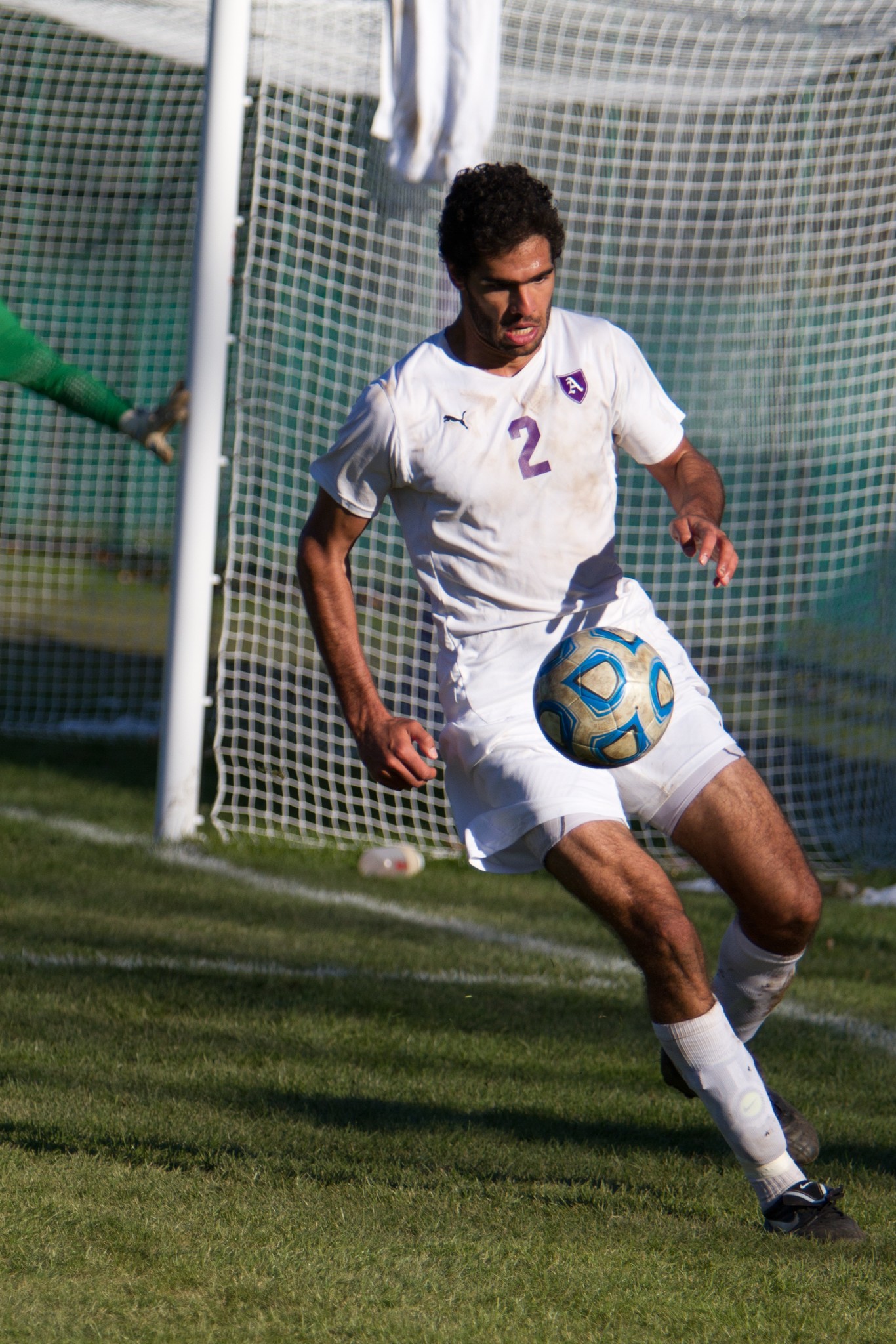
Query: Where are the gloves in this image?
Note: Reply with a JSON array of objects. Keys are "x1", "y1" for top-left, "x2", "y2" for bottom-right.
[{"x1": 119, "y1": 378, "x2": 189, "y2": 465}]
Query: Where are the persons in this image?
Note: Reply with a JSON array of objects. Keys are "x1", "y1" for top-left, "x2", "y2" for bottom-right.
[
  {"x1": 296, "y1": 162, "x2": 871, "y2": 1250},
  {"x1": 0, "y1": 297, "x2": 193, "y2": 462}
]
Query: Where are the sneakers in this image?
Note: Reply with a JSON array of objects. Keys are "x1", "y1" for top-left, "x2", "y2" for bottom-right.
[
  {"x1": 659, "y1": 1044, "x2": 820, "y2": 1165},
  {"x1": 763, "y1": 1180, "x2": 866, "y2": 1241}
]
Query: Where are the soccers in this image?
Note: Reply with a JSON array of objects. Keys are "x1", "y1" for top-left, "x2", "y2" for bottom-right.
[{"x1": 531, "y1": 625, "x2": 679, "y2": 770}]
